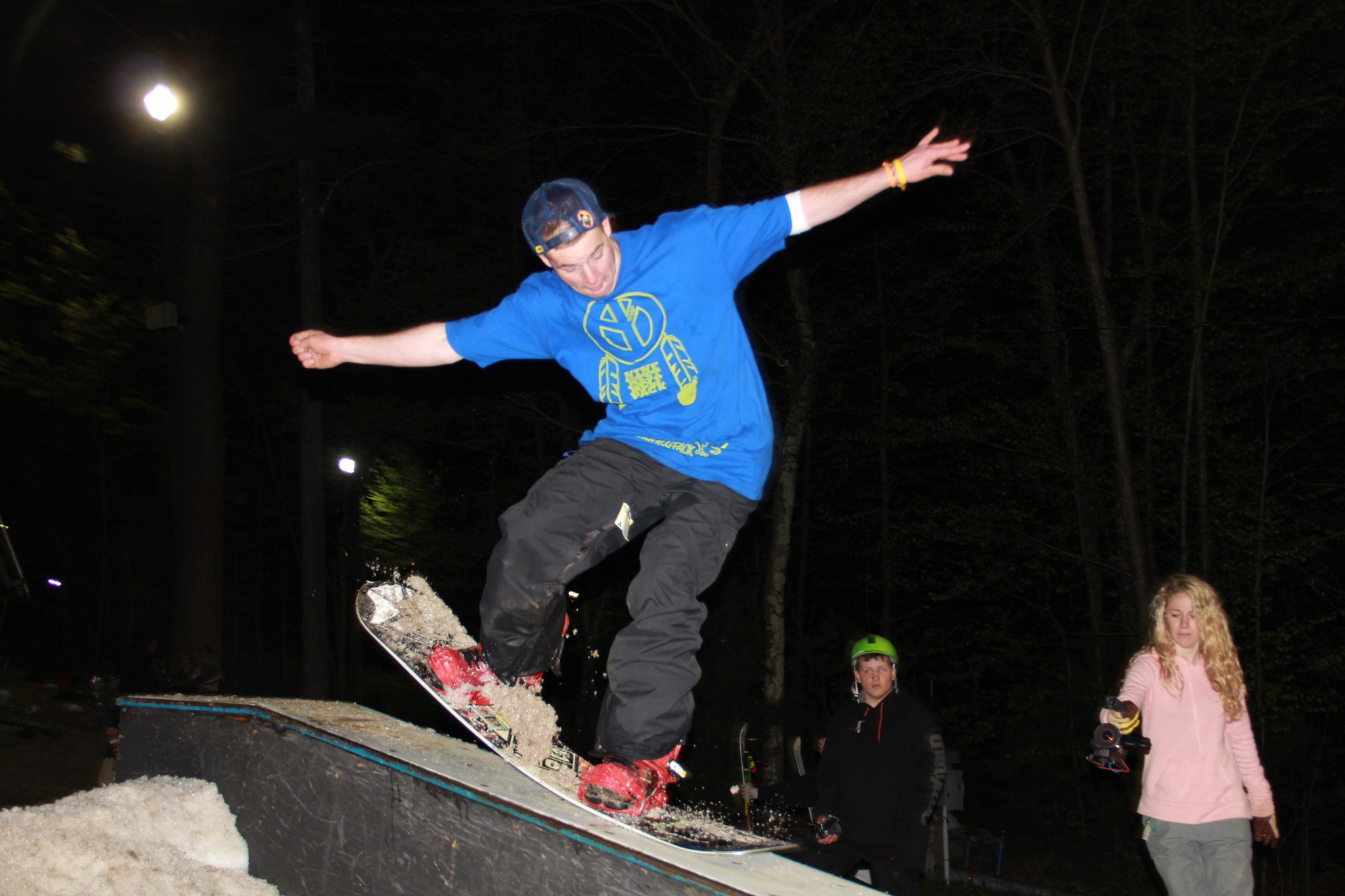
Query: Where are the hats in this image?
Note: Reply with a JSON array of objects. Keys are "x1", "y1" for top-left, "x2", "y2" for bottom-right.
[{"x1": 520, "y1": 178, "x2": 608, "y2": 255}]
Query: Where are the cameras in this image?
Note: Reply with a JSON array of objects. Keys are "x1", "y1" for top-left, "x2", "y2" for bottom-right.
[
  {"x1": 816, "y1": 815, "x2": 843, "y2": 839},
  {"x1": 1085, "y1": 722, "x2": 1152, "y2": 770}
]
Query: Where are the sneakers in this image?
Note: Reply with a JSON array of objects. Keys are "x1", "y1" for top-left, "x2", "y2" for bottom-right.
[
  {"x1": 429, "y1": 641, "x2": 542, "y2": 693},
  {"x1": 584, "y1": 739, "x2": 685, "y2": 819}
]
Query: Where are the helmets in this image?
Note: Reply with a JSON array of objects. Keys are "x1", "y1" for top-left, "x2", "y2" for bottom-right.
[{"x1": 850, "y1": 635, "x2": 897, "y2": 663}]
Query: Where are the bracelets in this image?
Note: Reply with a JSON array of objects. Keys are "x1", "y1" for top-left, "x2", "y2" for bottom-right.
[
  {"x1": 882, "y1": 161, "x2": 896, "y2": 188},
  {"x1": 893, "y1": 159, "x2": 906, "y2": 190}
]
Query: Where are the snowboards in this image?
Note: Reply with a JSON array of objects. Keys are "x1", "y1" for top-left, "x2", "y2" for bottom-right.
[
  {"x1": 792, "y1": 736, "x2": 821, "y2": 846},
  {"x1": 355, "y1": 582, "x2": 801, "y2": 855},
  {"x1": 739, "y1": 722, "x2": 755, "y2": 835}
]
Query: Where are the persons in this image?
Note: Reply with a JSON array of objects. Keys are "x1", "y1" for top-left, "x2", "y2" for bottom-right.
[
  {"x1": 740, "y1": 633, "x2": 964, "y2": 896},
  {"x1": 290, "y1": 129, "x2": 971, "y2": 816},
  {"x1": 98, "y1": 726, "x2": 119, "y2": 786},
  {"x1": 1099, "y1": 574, "x2": 1280, "y2": 896}
]
